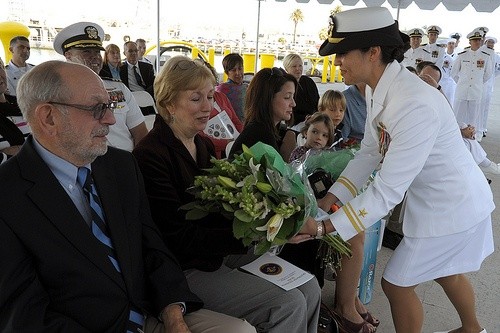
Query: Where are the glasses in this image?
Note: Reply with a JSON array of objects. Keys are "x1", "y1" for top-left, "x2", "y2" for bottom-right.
[
  {"x1": 268, "y1": 68, "x2": 286, "y2": 81},
  {"x1": 170, "y1": 58, "x2": 205, "y2": 72},
  {"x1": 45, "y1": 96, "x2": 118, "y2": 121}
]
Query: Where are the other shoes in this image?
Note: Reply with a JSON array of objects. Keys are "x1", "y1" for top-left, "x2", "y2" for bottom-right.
[
  {"x1": 483, "y1": 131, "x2": 487, "y2": 137},
  {"x1": 432, "y1": 326, "x2": 488, "y2": 333}
]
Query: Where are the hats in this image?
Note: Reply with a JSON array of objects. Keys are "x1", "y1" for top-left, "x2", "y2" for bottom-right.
[
  {"x1": 407, "y1": 28, "x2": 424, "y2": 38},
  {"x1": 451, "y1": 33, "x2": 461, "y2": 39},
  {"x1": 484, "y1": 36, "x2": 497, "y2": 44},
  {"x1": 436, "y1": 39, "x2": 447, "y2": 45},
  {"x1": 467, "y1": 30, "x2": 485, "y2": 40},
  {"x1": 426, "y1": 25, "x2": 442, "y2": 35},
  {"x1": 447, "y1": 38, "x2": 457, "y2": 43},
  {"x1": 52, "y1": 21, "x2": 106, "y2": 55},
  {"x1": 319, "y1": 6, "x2": 400, "y2": 57},
  {"x1": 474, "y1": 26, "x2": 489, "y2": 35}
]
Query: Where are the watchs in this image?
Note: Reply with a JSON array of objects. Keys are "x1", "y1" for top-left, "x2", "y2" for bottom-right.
[{"x1": 315, "y1": 221, "x2": 323, "y2": 240}]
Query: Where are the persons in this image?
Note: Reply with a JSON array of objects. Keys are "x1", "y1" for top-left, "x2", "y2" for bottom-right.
[
  {"x1": 0, "y1": 22, "x2": 382, "y2": 333},
  {"x1": 297, "y1": 6, "x2": 496, "y2": 333},
  {"x1": 401, "y1": 24, "x2": 500, "y2": 176}
]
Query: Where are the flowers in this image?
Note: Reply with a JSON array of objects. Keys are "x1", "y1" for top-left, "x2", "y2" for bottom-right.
[
  {"x1": 176, "y1": 144, "x2": 352, "y2": 276},
  {"x1": 341, "y1": 138, "x2": 360, "y2": 150}
]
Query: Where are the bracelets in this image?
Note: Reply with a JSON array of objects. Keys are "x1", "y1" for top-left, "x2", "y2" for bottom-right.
[{"x1": 322, "y1": 221, "x2": 328, "y2": 236}]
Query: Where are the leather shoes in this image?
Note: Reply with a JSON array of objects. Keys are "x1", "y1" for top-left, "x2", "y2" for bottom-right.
[{"x1": 478, "y1": 161, "x2": 500, "y2": 175}]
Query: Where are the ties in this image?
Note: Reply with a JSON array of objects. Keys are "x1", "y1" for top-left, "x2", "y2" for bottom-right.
[
  {"x1": 133, "y1": 66, "x2": 146, "y2": 91},
  {"x1": 75, "y1": 167, "x2": 144, "y2": 333}
]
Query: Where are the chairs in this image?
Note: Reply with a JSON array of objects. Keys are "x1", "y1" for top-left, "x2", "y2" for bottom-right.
[{"x1": 133, "y1": 91, "x2": 158, "y2": 128}]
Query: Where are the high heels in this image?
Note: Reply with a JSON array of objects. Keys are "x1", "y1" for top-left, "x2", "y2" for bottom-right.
[
  {"x1": 359, "y1": 312, "x2": 380, "y2": 326},
  {"x1": 331, "y1": 310, "x2": 377, "y2": 333}
]
